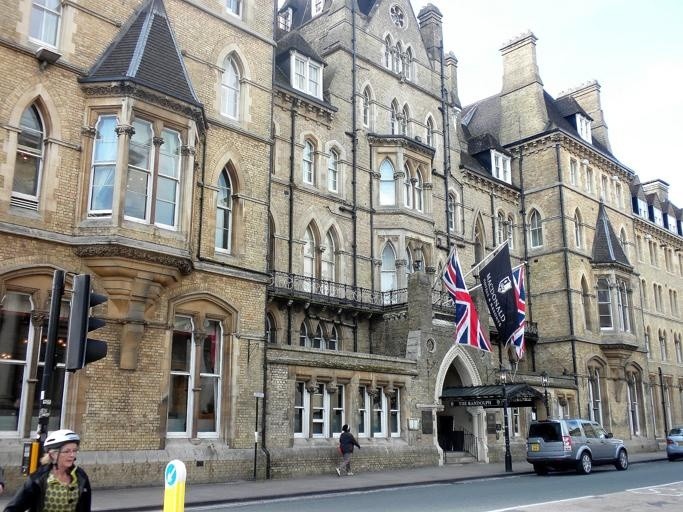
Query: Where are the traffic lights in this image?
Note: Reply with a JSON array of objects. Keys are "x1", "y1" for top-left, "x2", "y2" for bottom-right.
[{"x1": 64, "y1": 273, "x2": 108, "y2": 374}]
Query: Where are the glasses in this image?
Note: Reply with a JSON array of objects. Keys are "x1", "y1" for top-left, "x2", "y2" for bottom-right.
[{"x1": 54, "y1": 449, "x2": 80, "y2": 456}]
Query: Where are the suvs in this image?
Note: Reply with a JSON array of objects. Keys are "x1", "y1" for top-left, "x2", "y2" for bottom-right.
[{"x1": 525, "y1": 418, "x2": 629, "y2": 474}]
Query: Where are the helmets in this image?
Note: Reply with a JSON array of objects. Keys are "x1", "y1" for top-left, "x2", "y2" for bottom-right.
[{"x1": 43, "y1": 429, "x2": 80, "y2": 451}]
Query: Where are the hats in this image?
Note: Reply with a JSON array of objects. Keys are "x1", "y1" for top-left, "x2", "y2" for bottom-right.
[{"x1": 341, "y1": 425, "x2": 350, "y2": 432}]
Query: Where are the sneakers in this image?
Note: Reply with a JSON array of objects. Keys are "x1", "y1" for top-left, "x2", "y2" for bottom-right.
[
  {"x1": 347, "y1": 472, "x2": 353, "y2": 475},
  {"x1": 335, "y1": 467, "x2": 341, "y2": 475}
]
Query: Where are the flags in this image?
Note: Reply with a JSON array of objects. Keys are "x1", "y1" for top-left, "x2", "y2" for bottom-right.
[
  {"x1": 481, "y1": 244, "x2": 521, "y2": 349},
  {"x1": 507, "y1": 266, "x2": 527, "y2": 359},
  {"x1": 441, "y1": 248, "x2": 494, "y2": 352}
]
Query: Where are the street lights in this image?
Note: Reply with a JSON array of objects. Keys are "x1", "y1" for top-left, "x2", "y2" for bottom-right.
[
  {"x1": 540, "y1": 370, "x2": 550, "y2": 417},
  {"x1": 498, "y1": 367, "x2": 513, "y2": 472}
]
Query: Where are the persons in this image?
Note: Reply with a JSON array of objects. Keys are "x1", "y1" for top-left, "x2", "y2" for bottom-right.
[
  {"x1": 3, "y1": 430, "x2": 91, "y2": 512},
  {"x1": 335, "y1": 423, "x2": 360, "y2": 475},
  {"x1": 0, "y1": 468, "x2": 5, "y2": 493}
]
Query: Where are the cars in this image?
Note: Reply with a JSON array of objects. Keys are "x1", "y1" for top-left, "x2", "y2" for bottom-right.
[{"x1": 665, "y1": 427, "x2": 683, "y2": 462}]
